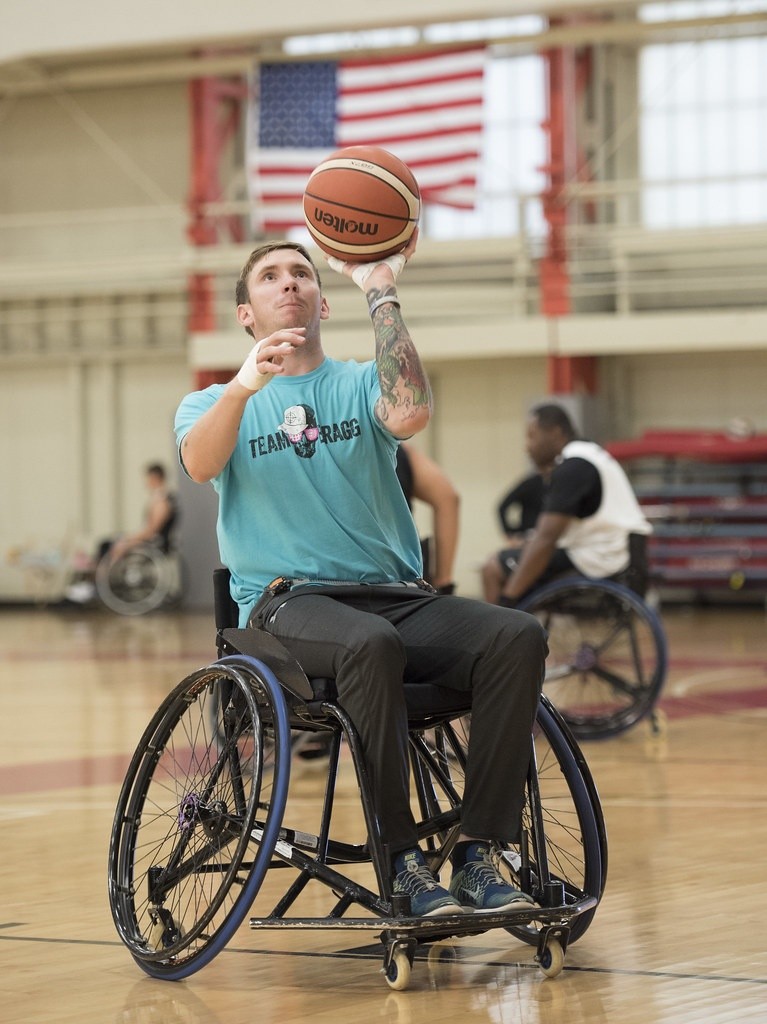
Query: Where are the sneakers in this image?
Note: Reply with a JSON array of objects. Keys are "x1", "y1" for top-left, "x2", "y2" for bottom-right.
[
  {"x1": 447, "y1": 839, "x2": 535, "y2": 914},
  {"x1": 390, "y1": 848, "x2": 463, "y2": 917}
]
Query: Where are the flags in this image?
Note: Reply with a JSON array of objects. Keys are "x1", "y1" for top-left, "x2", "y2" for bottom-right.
[{"x1": 249, "y1": 47, "x2": 483, "y2": 229}]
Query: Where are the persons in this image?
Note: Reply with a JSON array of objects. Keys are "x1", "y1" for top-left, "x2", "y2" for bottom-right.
[
  {"x1": 61, "y1": 465, "x2": 178, "y2": 605},
  {"x1": 175, "y1": 225, "x2": 548, "y2": 918},
  {"x1": 481, "y1": 402, "x2": 652, "y2": 617}
]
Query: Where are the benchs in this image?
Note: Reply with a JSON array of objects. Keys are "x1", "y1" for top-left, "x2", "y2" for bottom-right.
[{"x1": 605, "y1": 429, "x2": 767, "y2": 611}]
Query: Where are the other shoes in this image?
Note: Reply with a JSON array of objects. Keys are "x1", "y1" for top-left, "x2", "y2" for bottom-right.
[{"x1": 63, "y1": 579, "x2": 95, "y2": 603}]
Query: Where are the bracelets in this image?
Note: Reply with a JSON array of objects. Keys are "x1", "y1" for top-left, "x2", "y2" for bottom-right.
[{"x1": 370, "y1": 295, "x2": 401, "y2": 314}]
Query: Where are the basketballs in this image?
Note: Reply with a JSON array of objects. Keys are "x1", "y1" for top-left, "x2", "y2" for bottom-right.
[{"x1": 302, "y1": 144, "x2": 424, "y2": 265}]
